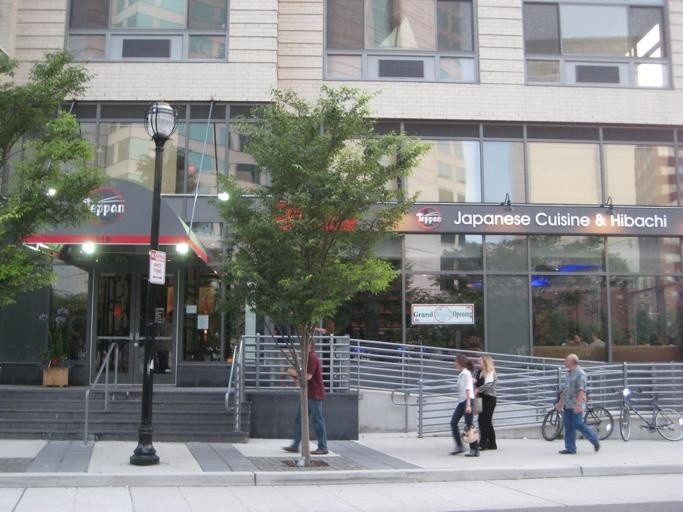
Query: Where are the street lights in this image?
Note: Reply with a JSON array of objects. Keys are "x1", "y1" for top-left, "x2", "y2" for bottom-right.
[{"x1": 129, "y1": 99, "x2": 181, "y2": 465}]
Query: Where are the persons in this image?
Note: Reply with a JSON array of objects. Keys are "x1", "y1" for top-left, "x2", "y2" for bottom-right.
[
  {"x1": 554, "y1": 352, "x2": 599, "y2": 453},
  {"x1": 535, "y1": 329, "x2": 680, "y2": 346},
  {"x1": 283, "y1": 337, "x2": 329, "y2": 454},
  {"x1": 350, "y1": 320, "x2": 484, "y2": 350},
  {"x1": 446, "y1": 354, "x2": 479, "y2": 457},
  {"x1": 469, "y1": 354, "x2": 498, "y2": 450}
]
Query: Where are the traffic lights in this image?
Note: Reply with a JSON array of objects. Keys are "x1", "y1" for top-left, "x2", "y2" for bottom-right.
[{"x1": 188, "y1": 167, "x2": 200, "y2": 192}]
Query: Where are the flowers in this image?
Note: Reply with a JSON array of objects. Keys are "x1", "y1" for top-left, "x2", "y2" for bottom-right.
[{"x1": 35, "y1": 305, "x2": 78, "y2": 370}]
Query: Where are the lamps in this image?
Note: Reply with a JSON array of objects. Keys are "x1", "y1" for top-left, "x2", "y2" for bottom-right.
[
  {"x1": 600, "y1": 196, "x2": 616, "y2": 215},
  {"x1": 500, "y1": 192, "x2": 512, "y2": 206}
]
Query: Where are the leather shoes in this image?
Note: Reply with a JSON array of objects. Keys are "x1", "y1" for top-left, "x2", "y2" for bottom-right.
[
  {"x1": 311, "y1": 448, "x2": 329, "y2": 454},
  {"x1": 282, "y1": 446, "x2": 298, "y2": 452}
]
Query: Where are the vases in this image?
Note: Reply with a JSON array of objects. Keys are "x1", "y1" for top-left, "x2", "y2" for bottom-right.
[{"x1": 42, "y1": 366, "x2": 70, "y2": 387}]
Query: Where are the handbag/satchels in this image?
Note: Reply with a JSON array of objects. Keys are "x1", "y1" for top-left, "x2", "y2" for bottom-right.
[
  {"x1": 472, "y1": 396, "x2": 483, "y2": 413},
  {"x1": 463, "y1": 422, "x2": 479, "y2": 444}
]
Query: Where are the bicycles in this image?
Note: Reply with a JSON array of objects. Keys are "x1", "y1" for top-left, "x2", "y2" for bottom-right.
[
  {"x1": 611, "y1": 384, "x2": 681, "y2": 443},
  {"x1": 539, "y1": 387, "x2": 616, "y2": 441}
]
